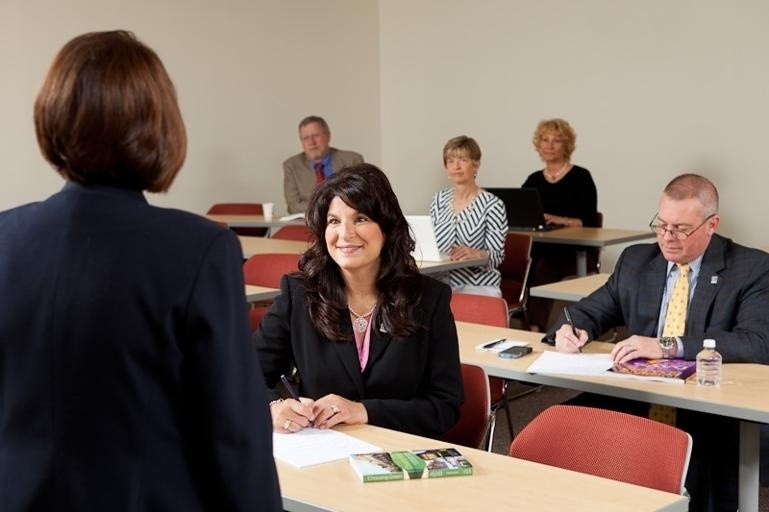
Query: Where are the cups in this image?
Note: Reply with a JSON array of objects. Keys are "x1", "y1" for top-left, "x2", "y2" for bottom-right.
[{"x1": 261, "y1": 203, "x2": 275, "y2": 220}]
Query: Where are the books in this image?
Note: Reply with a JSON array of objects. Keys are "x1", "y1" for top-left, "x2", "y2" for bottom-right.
[
  {"x1": 279, "y1": 213, "x2": 305, "y2": 222},
  {"x1": 605, "y1": 357, "x2": 696, "y2": 384},
  {"x1": 349, "y1": 448, "x2": 473, "y2": 483}
]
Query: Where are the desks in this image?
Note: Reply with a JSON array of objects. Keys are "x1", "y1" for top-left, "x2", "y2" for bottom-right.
[
  {"x1": 272, "y1": 423, "x2": 688, "y2": 512},
  {"x1": 528, "y1": 272, "x2": 612, "y2": 303},
  {"x1": 454, "y1": 320, "x2": 768, "y2": 512},
  {"x1": 505, "y1": 224, "x2": 658, "y2": 277}
]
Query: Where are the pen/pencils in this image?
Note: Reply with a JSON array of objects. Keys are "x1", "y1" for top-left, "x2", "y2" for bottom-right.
[
  {"x1": 280, "y1": 374, "x2": 301, "y2": 403},
  {"x1": 484, "y1": 339, "x2": 507, "y2": 348},
  {"x1": 564, "y1": 307, "x2": 582, "y2": 353}
]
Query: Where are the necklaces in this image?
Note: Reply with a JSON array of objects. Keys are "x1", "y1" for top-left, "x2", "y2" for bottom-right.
[
  {"x1": 545, "y1": 163, "x2": 567, "y2": 180},
  {"x1": 348, "y1": 304, "x2": 376, "y2": 334}
]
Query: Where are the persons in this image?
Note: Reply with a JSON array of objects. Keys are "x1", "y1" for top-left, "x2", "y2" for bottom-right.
[
  {"x1": 521, "y1": 118, "x2": 598, "y2": 331},
  {"x1": 429, "y1": 134, "x2": 509, "y2": 299},
  {"x1": 283, "y1": 115, "x2": 364, "y2": 214},
  {"x1": 254, "y1": 163, "x2": 465, "y2": 439},
  {"x1": 540, "y1": 173, "x2": 769, "y2": 512},
  {"x1": 0, "y1": 29, "x2": 283, "y2": 512}
]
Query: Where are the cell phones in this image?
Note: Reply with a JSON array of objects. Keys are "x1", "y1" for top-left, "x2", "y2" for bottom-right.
[{"x1": 498, "y1": 345, "x2": 532, "y2": 358}]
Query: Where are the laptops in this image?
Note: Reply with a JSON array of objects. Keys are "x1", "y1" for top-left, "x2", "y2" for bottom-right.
[{"x1": 483, "y1": 188, "x2": 564, "y2": 231}]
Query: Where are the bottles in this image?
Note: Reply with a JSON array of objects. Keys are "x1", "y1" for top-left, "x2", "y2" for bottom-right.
[{"x1": 696, "y1": 339, "x2": 722, "y2": 387}]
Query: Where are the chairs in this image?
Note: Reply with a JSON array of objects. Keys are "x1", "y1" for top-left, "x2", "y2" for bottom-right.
[{"x1": 509, "y1": 404, "x2": 696, "y2": 498}]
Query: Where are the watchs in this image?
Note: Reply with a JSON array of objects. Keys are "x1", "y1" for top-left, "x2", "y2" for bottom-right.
[{"x1": 658, "y1": 336, "x2": 674, "y2": 359}]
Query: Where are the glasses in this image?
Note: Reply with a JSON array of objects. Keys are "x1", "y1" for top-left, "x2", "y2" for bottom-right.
[{"x1": 648, "y1": 207, "x2": 718, "y2": 242}]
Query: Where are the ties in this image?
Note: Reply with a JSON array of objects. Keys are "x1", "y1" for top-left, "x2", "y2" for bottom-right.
[
  {"x1": 312, "y1": 162, "x2": 325, "y2": 188},
  {"x1": 648, "y1": 262, "x2": 691, "y2": 429}
]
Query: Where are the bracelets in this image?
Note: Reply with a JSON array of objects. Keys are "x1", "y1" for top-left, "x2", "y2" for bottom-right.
[{"x1": 568, "y1": 217, "x2": 571, "y2": 227}]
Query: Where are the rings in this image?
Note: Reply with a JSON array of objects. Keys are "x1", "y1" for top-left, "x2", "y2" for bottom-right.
[
  {"x1": 284, "y1": 420, "x2": 291, "y2": 429},
  {"x1": 330, "y1": 404, "x2": 337, "y2": 413}
]
